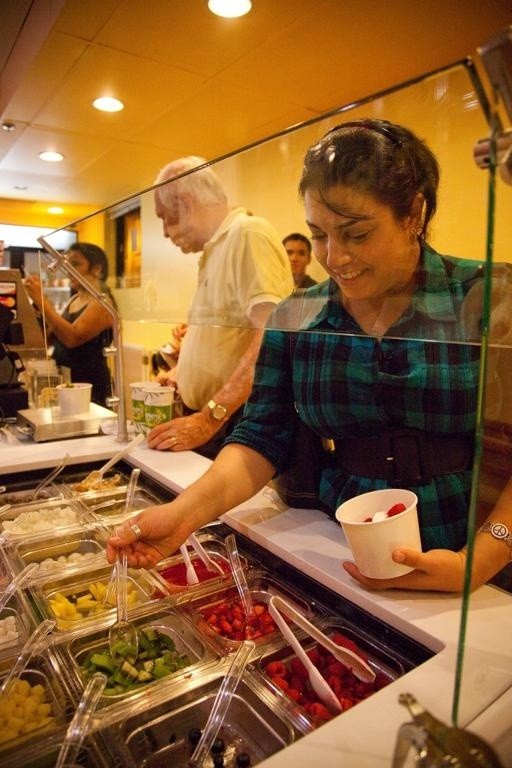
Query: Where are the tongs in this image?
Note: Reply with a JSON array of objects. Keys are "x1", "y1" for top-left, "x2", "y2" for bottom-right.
[
  {"x1": 177, "y1": 532, "x2": 225, "y2": 587},
  {"x1": 268, "y1": 595, "x2": 378, "y2": 713}
]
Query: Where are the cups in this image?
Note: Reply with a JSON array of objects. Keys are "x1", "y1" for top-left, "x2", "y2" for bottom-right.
[
  {"x1": 128, "y1": 381, "x2": 176, "y2": 439},
  {"x1": 55, "y1": 382, "x2": 93, "y2": 418},
  {"x1": 333, "y1": 487, "x2": 424, "y2": 581}
]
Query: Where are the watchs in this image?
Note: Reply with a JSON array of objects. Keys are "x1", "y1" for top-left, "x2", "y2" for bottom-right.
[{"x1": 206, "y1": 399, "x2": 231, "y2": 423}]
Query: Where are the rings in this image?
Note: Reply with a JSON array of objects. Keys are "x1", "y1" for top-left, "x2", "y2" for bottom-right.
[
  {"x1": 172, "y1": 436, "x2": 177, "y2": 445},
  {"x1": 130, "y1": 524, "x2": 143, "y2": 541}
]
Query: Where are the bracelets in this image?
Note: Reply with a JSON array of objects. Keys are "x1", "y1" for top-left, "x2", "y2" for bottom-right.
[{"x1": 478, "y1": 521, "x2": 512, "y2": 550}]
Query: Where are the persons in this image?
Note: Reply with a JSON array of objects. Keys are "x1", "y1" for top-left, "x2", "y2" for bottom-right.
[
  {"x1": 145, "y1": 153, "x2": 296, "y2": 469},
  {"x1": 280, "y1": 233, "x2": 319, "y2": 289},
  {"x1": 25, "y1": 242, "x2": 122, "y2": 410},
  {"x1": 150, "y1": 320, "x2": 189, "y2": 387},
  {"x1": 105, "y1": 116, "x2": 512, "y2": 597}
]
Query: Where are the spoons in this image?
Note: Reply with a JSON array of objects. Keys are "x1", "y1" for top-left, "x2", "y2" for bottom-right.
[{"x1": 110, "y1": 542, "x2": 142, "y2": 663}]
